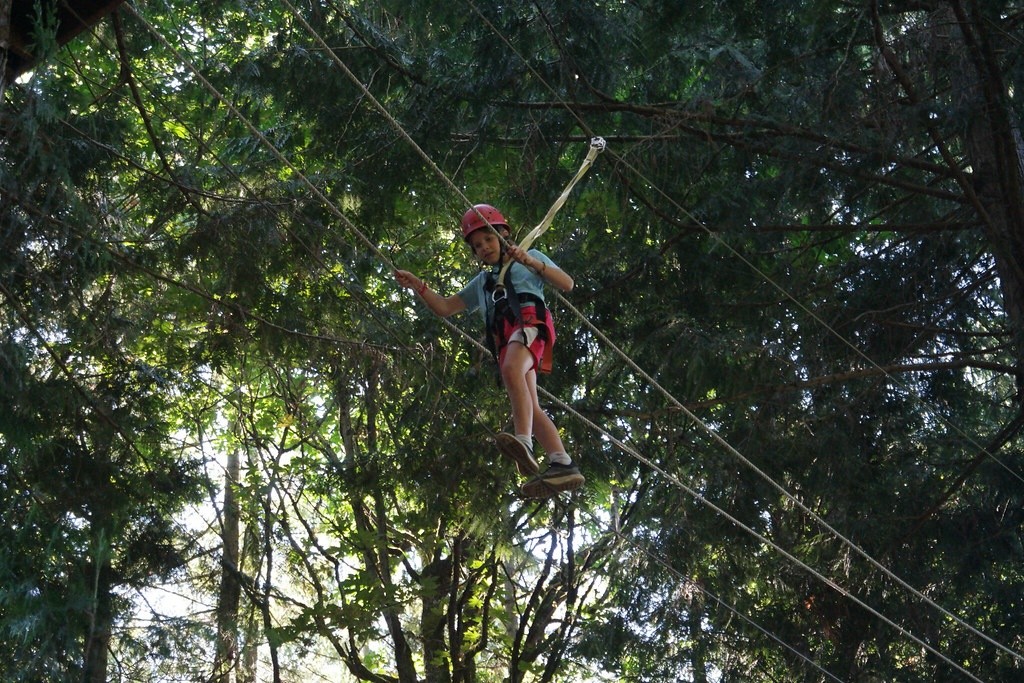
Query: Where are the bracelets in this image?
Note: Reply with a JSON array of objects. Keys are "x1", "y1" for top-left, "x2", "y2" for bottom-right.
[{"x1": 415, "y1": 278, "x2": 428, "y2": 296}]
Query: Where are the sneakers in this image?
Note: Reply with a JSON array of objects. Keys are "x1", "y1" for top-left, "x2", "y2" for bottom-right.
[
  {"x1": 522, "y1": 459, "x2": 585, "y2": 498},
  {"x1": 495, "y1": 432, "x2": 540, "y2": 477}
]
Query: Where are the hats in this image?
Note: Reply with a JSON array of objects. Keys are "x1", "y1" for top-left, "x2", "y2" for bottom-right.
[{"x1": 462, "y1": 204, "x2": 511, "y2": 243}]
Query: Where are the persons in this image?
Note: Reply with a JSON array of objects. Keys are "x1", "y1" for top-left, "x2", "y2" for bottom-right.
[
  {"x1": 397, "y1": 204, "x2": 585, "y2": 498},
  {"x1": 536, "y1": 262, "x2": 547, "y2": 275}
]
